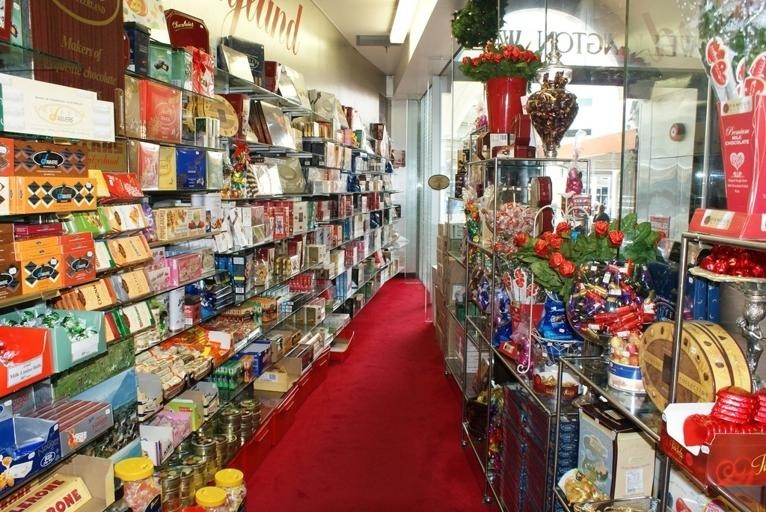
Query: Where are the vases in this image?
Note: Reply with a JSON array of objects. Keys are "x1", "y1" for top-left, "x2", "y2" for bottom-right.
[{"x1": 487, "y1": 78, "x2": 528, "y2": 133}]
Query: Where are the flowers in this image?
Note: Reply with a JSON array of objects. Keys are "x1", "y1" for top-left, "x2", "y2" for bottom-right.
[{"x1": 460, "y1": 42, "x2": 547, "y2": 92}]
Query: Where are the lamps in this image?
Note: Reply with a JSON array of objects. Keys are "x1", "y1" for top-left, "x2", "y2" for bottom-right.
[{"x1": 535, "y1": 32, "x2": 573, "y2": 86}]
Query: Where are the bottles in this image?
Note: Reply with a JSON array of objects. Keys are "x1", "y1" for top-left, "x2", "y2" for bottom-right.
[
  {"x1": 114, "y1": 457, "x2": 163, "y2": 512},
  {"x1": 195, "y1": 468, "x2": 247, "y2": 512}
]
